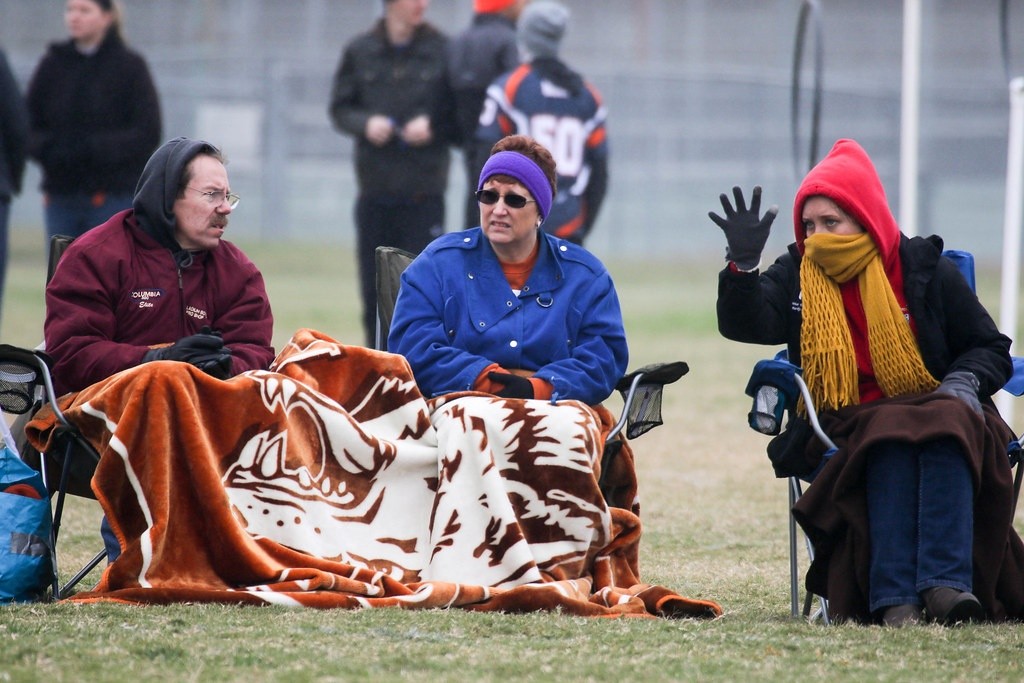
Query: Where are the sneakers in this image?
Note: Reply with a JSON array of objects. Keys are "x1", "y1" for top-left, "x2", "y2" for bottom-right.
[
  {"x1": 922, "y1": 587, "x2": 982, "y2": 627},
  {"x1": 882, "y1": 605, "x2": 927, "y2": 627}
]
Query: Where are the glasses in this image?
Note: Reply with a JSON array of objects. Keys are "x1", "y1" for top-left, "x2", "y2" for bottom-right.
[
  {"x1": 186, "y1": 186, "x2": 240, "y2": 209},
  {"x1": 475, "y1": 190, "x2": 536, "y2": 208}
]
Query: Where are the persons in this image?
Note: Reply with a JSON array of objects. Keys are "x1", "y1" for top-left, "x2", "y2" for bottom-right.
[
  {"x1": 328, "y1": 0, "x2": 470, "y2": 350},
  {"x1": 387, "y1": 135, "x2": 629, "y2": 407},
  {"x1": 0, "y1": 0, "x2": 161, "y2": 316},
  {"x1": 451, "y1": 0, "x2": 526, "y2": 174},
  {"x1": 708, "y1": 137, "x2": 1014, "y2": 629},
  {"x1": 462, "y1": 2, "x2": 610, "y2": 247},
  {"x1": 23, "y1": 137, "x2": 276, "y2": 565}
]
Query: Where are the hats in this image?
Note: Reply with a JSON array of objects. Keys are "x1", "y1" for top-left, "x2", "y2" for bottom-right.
[
  {"x1": 474, "y1": 0, "x2": 517, "y2": 15},
  {"x1": 517, "y1": 3, "x2": 566, "y2": 55}
]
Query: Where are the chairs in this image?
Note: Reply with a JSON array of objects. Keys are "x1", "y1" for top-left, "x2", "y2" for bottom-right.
[
  {"x1": 374, "y1": 245, "x2": 691, "y2": 496},
  {"x1": 743, "y1": 249, "x2": 1024, "y2": 625},
  {"x1": 0, "y1": 233, "x2": 107, "y2": 600}
]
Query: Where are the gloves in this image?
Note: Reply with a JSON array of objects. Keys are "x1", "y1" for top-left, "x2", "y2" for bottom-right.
[
  {"x1": 708, "y1": 185, "x2": 778, "y2": 270},
  {"x1": 933, "y1": 372, "x2": 985, "y2": 423},
  {"x1": 140, "y1": 326, "x2": 232, "y2": 378},
  {"x1": 488, "y1": 372, "x2": 534, "y2": 399}
]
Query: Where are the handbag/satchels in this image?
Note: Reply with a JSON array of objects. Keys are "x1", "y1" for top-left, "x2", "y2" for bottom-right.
[{"x1": 0, "y1": 406, "x2": 52, "y2": 604}]
